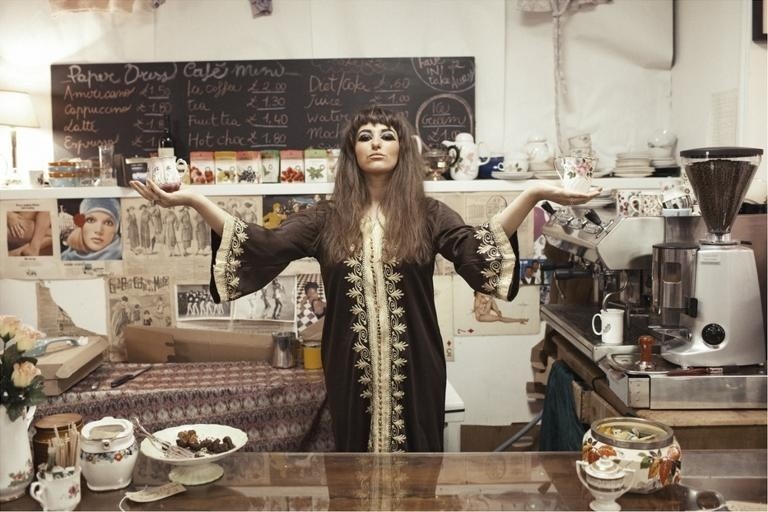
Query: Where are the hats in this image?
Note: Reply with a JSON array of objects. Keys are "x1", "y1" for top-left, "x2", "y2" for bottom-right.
[{"x1": 77, "y1": 198, "x2": 122, "y2": 225}]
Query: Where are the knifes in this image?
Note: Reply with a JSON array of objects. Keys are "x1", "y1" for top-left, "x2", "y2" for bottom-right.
[{"x1": 110, "y1": 366, "x2": 153, "y2": 388}]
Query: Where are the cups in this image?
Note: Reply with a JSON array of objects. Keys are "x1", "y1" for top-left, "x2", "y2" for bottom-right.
[
  {"x1": 592, "y1": 308, "x2": 625, "y2": 344},
  {"x1": 571, "y1": 149, "x2": 596, "y2": 158},
  {"x1": 30, "y1": 171, "x2": 43, "y2": 188},
  {"x1": 30, "y1": 467, "x2": 82, "y2": 512},
  {"x1": 568, "y1": 133, "x2": 591, "y2": 148},
  {"x1": 98, "y1": 144, "x2": 114, "y2": 186},
  {"x1": 498, "y1": 153, "x2": 529, "y2": 173},
  {"x1": 80, "y1": 168, "x2": 92, "y2": 187},
  {"x1": 105, "y1": 168, "x2": 117, "y2": 187}
]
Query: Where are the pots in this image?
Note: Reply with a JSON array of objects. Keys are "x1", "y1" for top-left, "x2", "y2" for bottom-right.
[{"x1": 581, "y1": 417, "x2": 681, "y2": 495}]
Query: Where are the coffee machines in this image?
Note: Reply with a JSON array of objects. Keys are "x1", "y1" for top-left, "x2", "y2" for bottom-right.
[
  {"x1": 597, "y1": 146, "x2": 768, "y2": 410},
  {"x1": 539, "y1": 201, "x2": 702, "y2": 362}
]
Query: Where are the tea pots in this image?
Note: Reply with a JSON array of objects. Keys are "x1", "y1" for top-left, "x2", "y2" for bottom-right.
[
  {"x1": 422, "y1": 145, "x2": 459, "y2": 181},
  {"x1": 272, "y1": 332, "x2": 296, "y2": 369},
  {"x1": 442, "y1": 133, "x2": 490, "y2": 181},
  {"x1": 576, "y1": 454, "x2": 636, "y2": 512},
  {"x1": 647, "y1": 130, "x2": 678, "y2": 157},
  {"x1": 81, "y1": 416, "x2": 139, "y2": 491},
  {"x1": 523, "y1": 137, "x2": 553, "y2": 171},
  {"x1": 553, "y1": 156, "x2": 598, "y2": 193},
  {"x1": 126, "y1": 156, "x2": 188, "y2": 192}
]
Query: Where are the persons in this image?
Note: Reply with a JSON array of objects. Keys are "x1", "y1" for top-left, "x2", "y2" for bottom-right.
[
  {"x1": 301, "y1": 279, "x2": 326, "y2": 317},
  {"x1": 178, "y1": 287, "x2": 226, "y2": 315},
  {"x1": 241, "y1": 276, "x2": 287, "y2": 320},
  {"x1": 128, "y1": 106, "x2": 604, "y2": 453},
  {"x1": 9, "y1": 212, "x2": 54, "y2": 257},
  {"x1": 61, "y1": 199, "x2": 123, "y2": 258},
  {"x1": 471, "y1": 288, "x2": 530, "y2": 327},
  {"x1": 112, "y1": 292, "x2": 168, "y2": 336},
  {"x1": 522, "y1": 261, "x2": 542, "y2": 286},
  {"x1": 126, "y1": 200, "x2": 260, "y2": 258}
]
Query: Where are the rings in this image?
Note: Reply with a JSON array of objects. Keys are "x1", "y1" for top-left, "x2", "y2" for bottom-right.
[{"x1": 149, "y1": 198, "x2": 157, "y2": 207}]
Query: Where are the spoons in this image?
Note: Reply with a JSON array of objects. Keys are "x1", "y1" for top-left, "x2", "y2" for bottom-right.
[{"x1": 23, "y1": 339, "x2": 78, "y2": 357}]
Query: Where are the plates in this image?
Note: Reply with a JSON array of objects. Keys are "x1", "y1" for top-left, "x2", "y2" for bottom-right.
[
  {"x1": 491, "y1": 171, "x2": 532, "y2": 181},
  {"x1": 613, "y1": 152, "x2": 655, "y2": 177},
  {"x1": 140, "y1": 423, "x2": 249, "y2": 486},
  {"x1": 535, "y1": 171, "x2": 560, "y2": 179},
  {"x1": 651, "y1": 157, "x2": 681, "y2": 177},
  {"x1": 591, "y1": 170, "x2": 606, "y2": 178}
]
